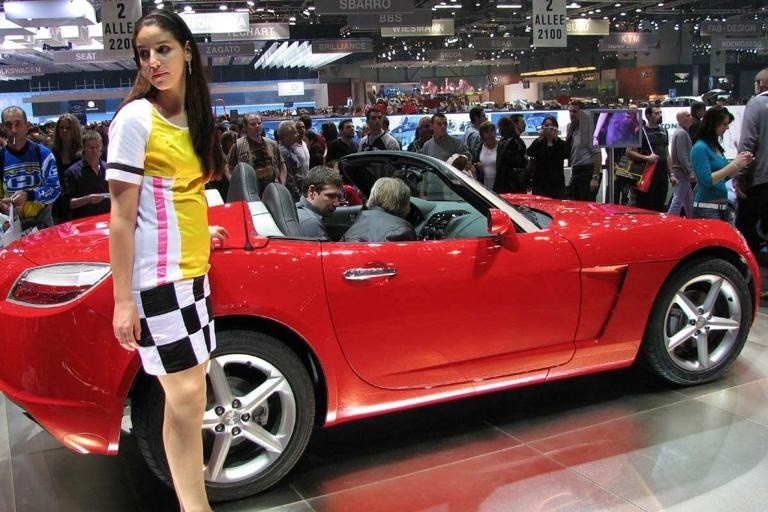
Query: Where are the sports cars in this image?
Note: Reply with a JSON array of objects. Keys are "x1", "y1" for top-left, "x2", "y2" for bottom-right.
[
  {"x1": 0, "y1": 149, "x2": 763, "y2": 506},
  {"x1": 222, "y1": 363, "x2": 765, "y2": 512}
]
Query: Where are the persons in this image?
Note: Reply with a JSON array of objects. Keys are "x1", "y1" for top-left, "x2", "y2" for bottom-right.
[
  {"x1": 0, "y1": 73, "x2": 766, "y2": 254},
  {"x1": 104, "y1": 9, "x2": 230, "y2": 512}
]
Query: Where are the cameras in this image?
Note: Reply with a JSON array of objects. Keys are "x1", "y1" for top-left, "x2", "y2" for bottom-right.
[{"x1": 544, "y1": 127, "x2": 551, "y2": 134}]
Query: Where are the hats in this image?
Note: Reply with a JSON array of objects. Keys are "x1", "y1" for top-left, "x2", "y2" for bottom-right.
[{"x1": 479, "y1": 120, "x2": 494, "y2": 130}]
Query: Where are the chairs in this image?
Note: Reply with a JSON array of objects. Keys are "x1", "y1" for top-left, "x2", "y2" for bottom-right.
[
  {"x1": 261, "y1": 182, "x2": 306, "y2": 237},
  {"x1": 226, "y1": 163, "x2": 260, "y2": 202}
]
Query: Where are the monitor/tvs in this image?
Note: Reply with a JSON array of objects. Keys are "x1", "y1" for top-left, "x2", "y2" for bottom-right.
[
  {"x1": 591, "y1": 109, "x2": 642, "y2": 147},
  {"x1": 579, "y1": 110, "x2": 591, "y2": 147}
]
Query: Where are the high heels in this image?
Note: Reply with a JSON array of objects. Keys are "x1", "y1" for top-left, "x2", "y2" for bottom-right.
[{"x1": 0, "y1": 198, "x2": 38, "y2": 251}]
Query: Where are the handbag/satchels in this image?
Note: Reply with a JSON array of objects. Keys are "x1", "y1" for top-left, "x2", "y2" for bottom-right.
[
  {"x1": 523, "y1": 157, "x2": 537, "y2": 177},
  {"x1": 614, "y1": 151, "x2": 659, "y2": 193}
]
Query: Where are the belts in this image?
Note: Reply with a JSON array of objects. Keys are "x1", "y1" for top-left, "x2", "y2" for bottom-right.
[
  {"x1": 692, "y1": 201, "x2": 728, "y2": 211},
  {"x1": 572, "y1": 162, "x2": 595, "y2": 174}
]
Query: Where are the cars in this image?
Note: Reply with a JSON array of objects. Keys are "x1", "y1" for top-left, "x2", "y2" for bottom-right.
[
  {"x1": 468, "y1": 89, "x2": 729, "y2": 112},
  {"x1": 371, "y1": 93, "x2": 451, "y2": 113}
]
